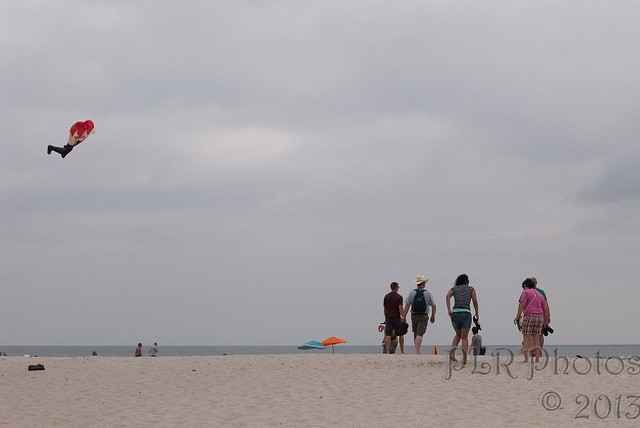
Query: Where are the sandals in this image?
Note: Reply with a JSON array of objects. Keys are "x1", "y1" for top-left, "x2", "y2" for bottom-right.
[{"x1": 473, "y1": 316, "x2": 481, "y2": 330}]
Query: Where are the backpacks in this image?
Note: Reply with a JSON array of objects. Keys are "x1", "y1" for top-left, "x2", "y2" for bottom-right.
[{"x1": 411, "y1": 286, "x2": 429, "y2": 313}]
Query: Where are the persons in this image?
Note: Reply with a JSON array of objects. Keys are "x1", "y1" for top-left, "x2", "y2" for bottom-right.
[
  {"x1": 469, "y1": 327, "x2": 482, "y2": 356},
  {"x1": 518, "y1": 278, "x2": 551, "y2": 356},
  {"x1": 135, "y1": 343, "x2": 143, "y2": 358},
  {"x1": 513, "y1": 279, "x2": 548, "y2": 364},
  {"x1": 382, "y1": 282, "x2": 407, "y2": 354},
  {"x1": 149, "y1": 341, "x2": 160, "y2": 357},
  {"x1": 401, "y1": 276, "x2": 436, "y2": 354},
  {"x1": 446, "y1": 273, "x2": 479, "y2": 364}
]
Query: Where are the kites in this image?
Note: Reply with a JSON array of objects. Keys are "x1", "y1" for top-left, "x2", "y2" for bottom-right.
[{"x1": 46, "y1": 119, "x2": 96, "y2": 159}]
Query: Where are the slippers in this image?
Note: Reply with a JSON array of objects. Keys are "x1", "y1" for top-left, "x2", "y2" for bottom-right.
[
  {"x1": 543, "y1": 324, "x2": 548, "y2": 336},
  {"x1": 545, "y1": 322, "x2": 554, "y2": 333}
]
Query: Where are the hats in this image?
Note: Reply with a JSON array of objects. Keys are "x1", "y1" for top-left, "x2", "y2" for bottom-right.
[{"x1": 412, "y1": 275, "x2": 429, "y2": 285}]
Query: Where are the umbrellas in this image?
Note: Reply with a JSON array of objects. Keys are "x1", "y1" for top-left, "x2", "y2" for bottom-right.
[
  {"x1": 297, "y1": 340, "x2": 326, "y2": 351},
  {"x1": 322, "y1": 336, "x2": 347, "y2": 353}
]
